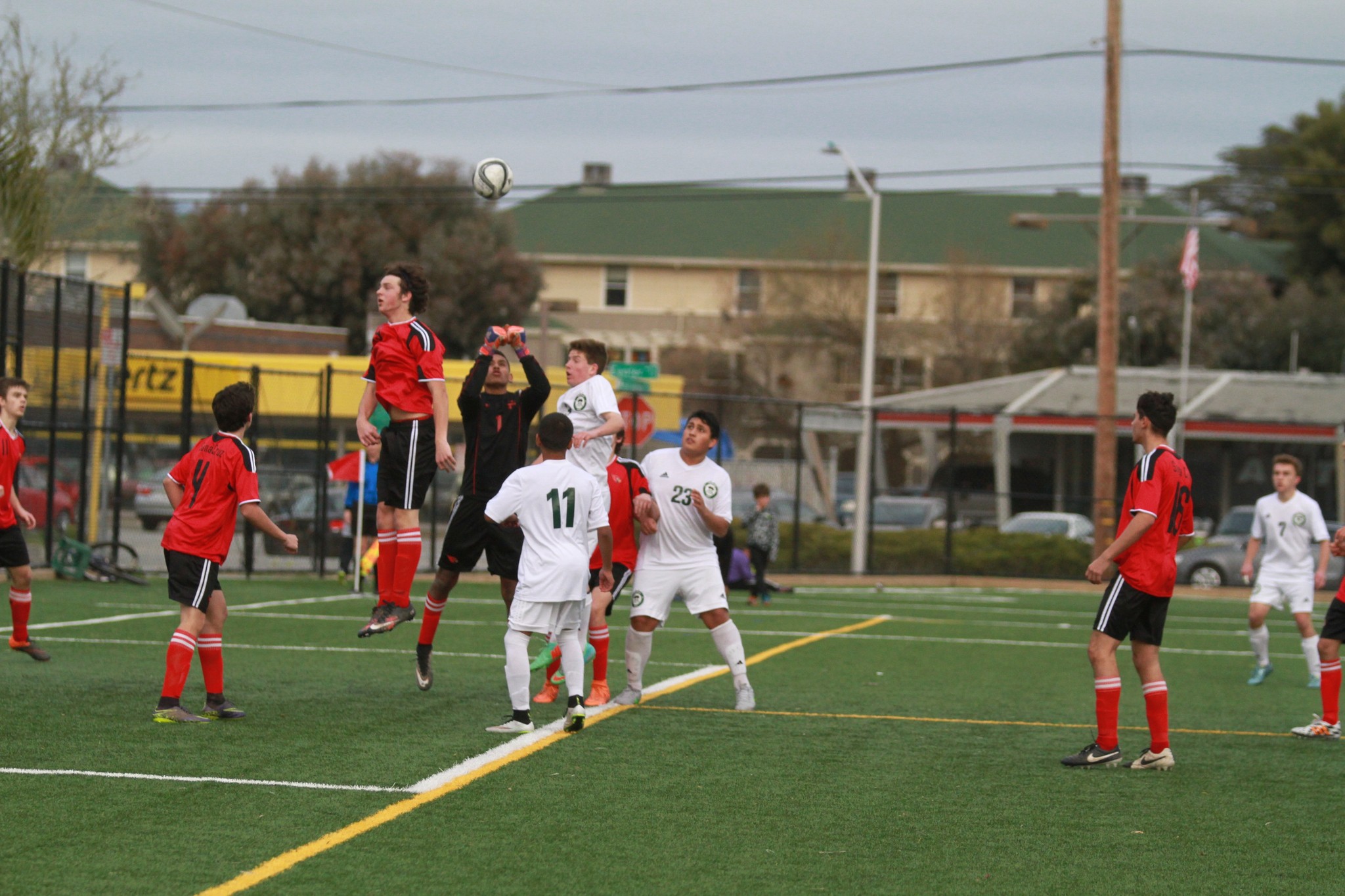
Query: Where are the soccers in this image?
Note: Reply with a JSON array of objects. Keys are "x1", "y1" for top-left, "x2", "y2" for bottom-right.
[{"x1": 472, "y1": 157, "x2": 514, "y2": 201}]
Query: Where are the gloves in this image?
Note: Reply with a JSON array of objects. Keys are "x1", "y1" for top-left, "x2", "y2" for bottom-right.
[
  {"x1": 480, "y1": 326, "x2": 508, "y2": 355},
  {"x1": 504, "y1": 323, "x2": 530, "y2": 358}
]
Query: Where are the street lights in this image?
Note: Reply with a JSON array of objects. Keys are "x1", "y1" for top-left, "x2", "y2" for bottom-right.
[{"x1": 819, "y1": 139, "x2": 882, "y2": 575}]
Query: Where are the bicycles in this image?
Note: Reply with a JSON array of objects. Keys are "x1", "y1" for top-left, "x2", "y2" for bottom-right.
[{"x1": 50, "y1": 524, "x2": 149, "y2": 587}]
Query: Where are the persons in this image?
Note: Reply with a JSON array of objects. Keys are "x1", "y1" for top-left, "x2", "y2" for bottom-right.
[
  {"x1": 712, "y1": 485, "x2": 779, "y2": 603},
  {"x1": 154, "y1": 381, "x2": 297, "y2": 725},
  {"x1": 1241, "y1": 454, "x2": 1323, "y2": 689},
  {"x1": 415, "y1": 325, "x2": 551, "y2": 692},
  {"x1": 1290, "y1": 528, "x2": 1345, "y2": 737},
  {"x1": 484, "y1": 413, "x2": 614, "y2": 733},
  {"x1": 1061, "y1": 390, "x2": 1193, "y2": 770},
  {"x1": 0, "y1": 376, "x2": 50, "y2": 662},
  {"x1": 528, "y1": 339, "x2": 625, "y2": 683},
  {"x1": 616, "y1": 410, "x2": 754, "y2": 711},
  {"x1": 339, "y1": 443, "x2": 381, "y2": 597},
  {"x1": 532, "y1": 427, "x2": 659, "y2": 707},
  {"x1": 356, "y1": 263, "x2": 456, "y2": 639}
]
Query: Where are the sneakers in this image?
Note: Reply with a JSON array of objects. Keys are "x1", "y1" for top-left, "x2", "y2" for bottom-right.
[
  {"x1": 532, "y1": 685, "x2": 559, "y2": 703},
  {"x1": 529, "y1": 642, "x2": 561, "y2": 672},
  {"x1": 151, "y1": 705, "x2": 211, "y2": 724},
  {"x1": 358, "y1": 605, "x2": 385, "y2": 638},
  {"x1": 9, "y1": 635, "x2": 50, "y2": 660},
  {"x1": 367, "y1": 600, "x2": 416, "y2": 634},
  {"x1": 735, "y1": 689, "x2": 756, "y2": 711},
  {"x1": 1248, "y1": 665, "x2": 1272, "y2": 685},
  {"x1": 585, "y1": 686, "x2": 610, "y2": 707},
  {"x1": 485, "y1": 715, "x2": 535, "y2": 733},
  {"x1": 551, "y1": 644, "x2": 595, "y2": 685},
  {"x1": 1308, "y1": 677, "x2": 1321, "y2": 687},
  {"x1": 202, "y1": 700, "x2": 245, "y2": 720},
  {"x1": 1124, "y1": 747, "x2": 1177, "y2": 772},
  {"x1": 1060, "y1": 743, "x2": 1121, "y2": 767},
  {"x1": 415, "y1": 646, "x2": 433, "y2": 690},
  {"x1": 614, "y1": 688, "x2": 639, "y2": 705},
  {"x1": 1291, "y1": 714, "x2": 1342, "y2": 740},
  {"x1": 563, "y1": 704, "x2": 586, "y2": 732}
]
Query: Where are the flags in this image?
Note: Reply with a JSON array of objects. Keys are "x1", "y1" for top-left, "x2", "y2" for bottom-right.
[{"x1": 1180, "y1": 227, "x2": 1200, "y2": 288}]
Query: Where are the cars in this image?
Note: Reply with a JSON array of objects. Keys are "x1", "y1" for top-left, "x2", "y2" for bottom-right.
[
  {"x1": 1203, "y1": 505, "x2": 1256, "y2": 544},
  {"x1": 16, "y1": 451, "x2": 351, "y2": 556},
  {"x1": 839, "y1": 494, "x2": 963, "y2": 532},
  {"x1": 997, "y1": 510, "x2": 1096, "y2": 547},
  {"x1": 1175, "y1": 519, "x2": 1345, "y2": 592},
  {"x1": 731, "y1": 486, "x2": 831, "y2": 526}
]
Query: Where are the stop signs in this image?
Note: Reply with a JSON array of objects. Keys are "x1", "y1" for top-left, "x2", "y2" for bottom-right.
[{"x1": 615, "y1": 395, "x2": 656, "y2": 446}]
